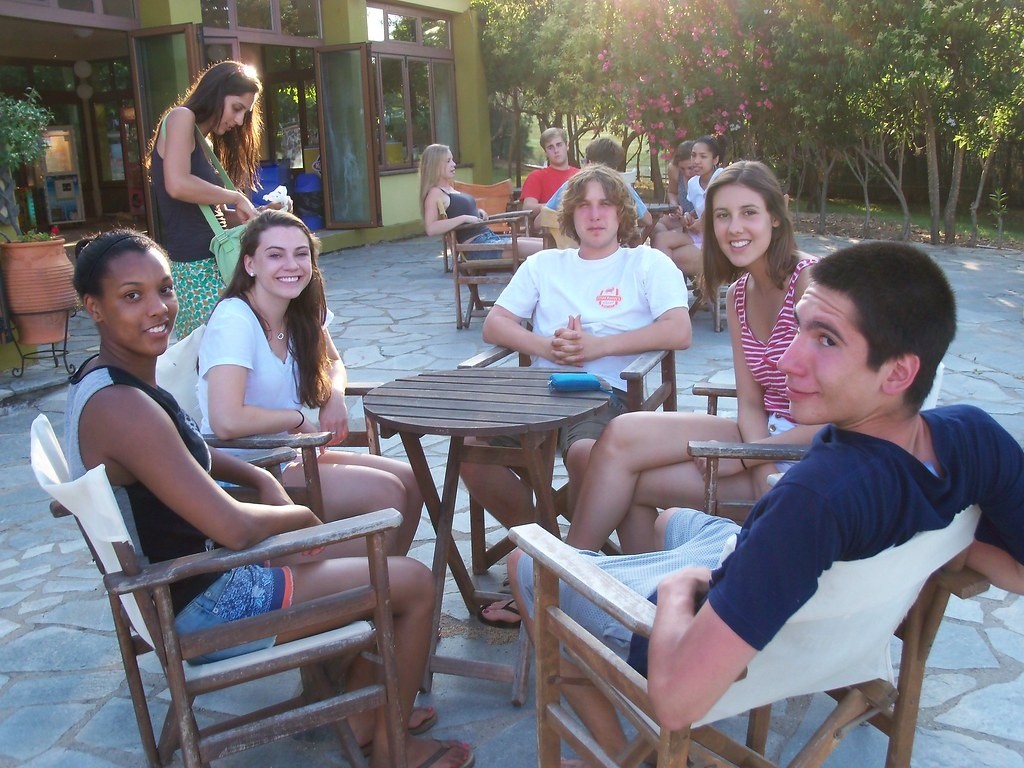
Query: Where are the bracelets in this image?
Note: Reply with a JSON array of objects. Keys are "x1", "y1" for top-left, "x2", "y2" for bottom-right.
[
  {"x1": 294, "y1": 410, "x2": 304, "y2": 429},
  {"x1": 741, "y1": 459, "x2": 747, "y2": 470}
]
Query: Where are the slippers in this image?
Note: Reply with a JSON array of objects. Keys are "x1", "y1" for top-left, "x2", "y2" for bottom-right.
[
  {"x1": 409, "y1": 738, "x2": 475, "y2": 768},
  {"x1": 339, "y1": 702, "x2": 436, "y2": 761},
  {"x1": 476, "y1": 596, "x2": 522, "y2": 630}
]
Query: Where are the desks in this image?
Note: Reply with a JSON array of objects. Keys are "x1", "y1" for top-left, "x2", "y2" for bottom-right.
[
  {"x1": 362, "y1": 366, "x2": 612, "y2": 706},
  {"x1": 640, "y1": 203, "x2": 678, "y2": 245}
]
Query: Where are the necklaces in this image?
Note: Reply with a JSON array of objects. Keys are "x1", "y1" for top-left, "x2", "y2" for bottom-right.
[{"x1": 277, "y1": 332, "x2": 284, "y2": 340}]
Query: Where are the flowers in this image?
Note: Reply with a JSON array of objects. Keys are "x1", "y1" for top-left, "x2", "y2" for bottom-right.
[{"x1": 16, "y1": 227, "x2": 58, "y2": 242}]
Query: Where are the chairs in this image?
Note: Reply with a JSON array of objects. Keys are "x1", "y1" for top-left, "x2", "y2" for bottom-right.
[
  {"x1": 710, "y1": 194, "x2": 789, "y2": 332},
  {"x1": 155, "y1": 323, "x2": 384, "y2": 522},
  {"x1": 443, "y1": 178, "x2": 513, "y2": 272},
  {"x1": 436, "y1": 199, "x2": 533, "y2": 330},
  {"x1": 688, "y1": 362, "x2": 944, "y2": 516},
  {"x1": 457, "y1": 321, "x2": 678, "y2": 576},
  {"x1": 541, "y1": 205, "x2": 637, "y2": 250},
  {"x1": 29, "y1": 414, "x2": 409, "y2": 768},
  {"x1": 508, "y1": 504, "x2": 990, "y2": 768}
]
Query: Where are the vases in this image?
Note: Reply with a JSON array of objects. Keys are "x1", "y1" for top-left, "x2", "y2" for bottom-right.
[{"x1": 0, "y1": 236, "x2": 77, "y2": 345}]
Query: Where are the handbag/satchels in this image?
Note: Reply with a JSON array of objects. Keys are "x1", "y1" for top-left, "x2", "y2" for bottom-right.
[{"x1": 208, "y1": 223, "x2": 250, "y2": 287}]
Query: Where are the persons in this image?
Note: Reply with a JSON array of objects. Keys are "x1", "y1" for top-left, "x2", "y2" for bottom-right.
[
  {"x1": 460, "y1": 163, "x2": 693, "y2": 530},
  {"x1": 506, "y1": 241, "x2": 1024, "y2": 768},
  {"x1": 197, "y1": 210, "x2": 425, "y2": 556},
  {"x1": 419, "y1": 144, "x2": 544, "y2": 260},
  {"x1": 519, "y1": 127, "x2": 582, "y2": 219},
  {"x1": 146, "y1": 62, "x2": 294, "y2": 342},
  {"x1": 533, "y1": 138, "x2": 653, "y2": 229},
  {"x1": 652, "y1": 134, "x2": 728, "y2": 276},
  {"x1": 647, "y1": 140, "x2": 698, "y2": 239},
  {"x1": 62, "y1": 226, "x2": 475, "y2": 768},
  {"x1": 477, "y1": 158, "x2": 828, "y2": 629}
]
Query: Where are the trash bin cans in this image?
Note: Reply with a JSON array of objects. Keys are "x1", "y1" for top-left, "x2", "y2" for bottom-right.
[
  {"x1": 251, "y1": 160, "x2": 280, "y2": 207},
  {"x1": 294, "y1": 173, "x2": 324, "y2": 231}
]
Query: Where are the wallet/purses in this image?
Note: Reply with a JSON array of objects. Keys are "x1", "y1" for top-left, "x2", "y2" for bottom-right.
[{"x1": 548, "y1": 372, "x2": 619, "y2": 408}]
